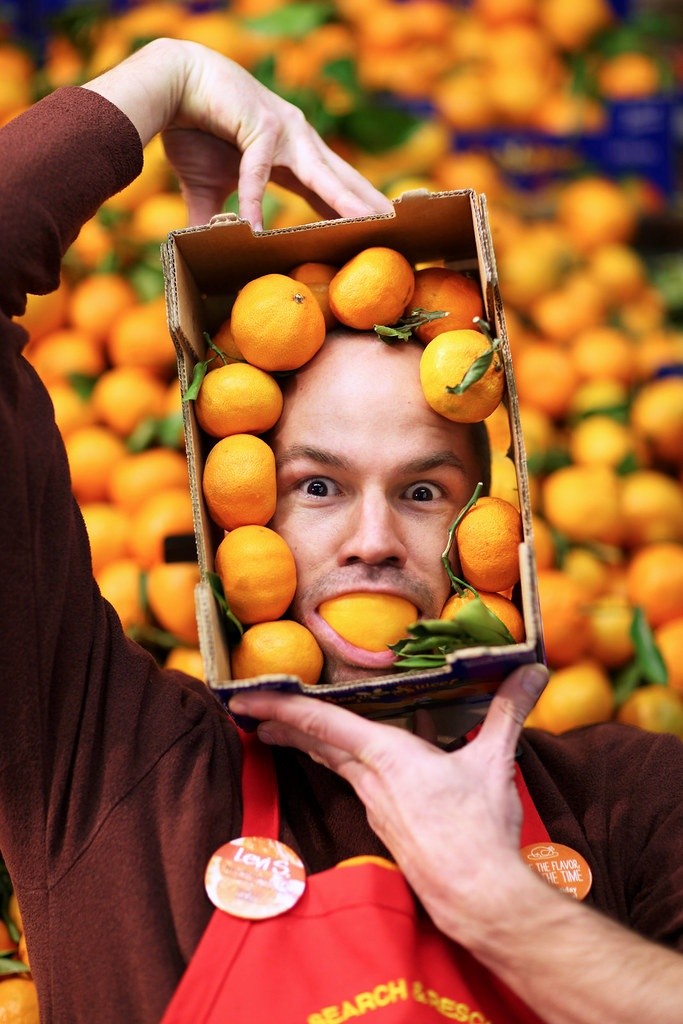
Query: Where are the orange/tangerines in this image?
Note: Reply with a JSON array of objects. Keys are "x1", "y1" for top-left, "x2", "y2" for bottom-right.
[
  {"x1": 0, "y1": 1, "x2": 682, "y2": 740},
  {"x1": 0, "y1": 891, "x2": 40, "y2": 1024}
]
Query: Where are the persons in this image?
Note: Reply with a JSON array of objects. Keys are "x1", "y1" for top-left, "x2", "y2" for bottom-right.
[{"x1": 0, "y1": 36, "x2": 683, "y2": 1024}]
